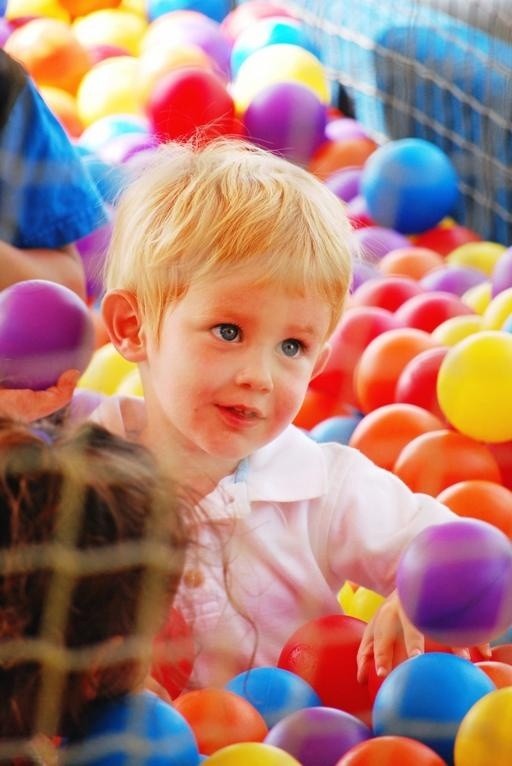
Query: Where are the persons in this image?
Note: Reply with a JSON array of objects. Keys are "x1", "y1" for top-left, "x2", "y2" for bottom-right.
[
  {"x1": 0, "y1": 48, "x2": 110, "y2": 303},
  {"x1": 2, "y1": 143, "x2": 492, "y2": 698},
  {"x1": 0, "y1": 415, "x2": 195, "y2": 766}
]
